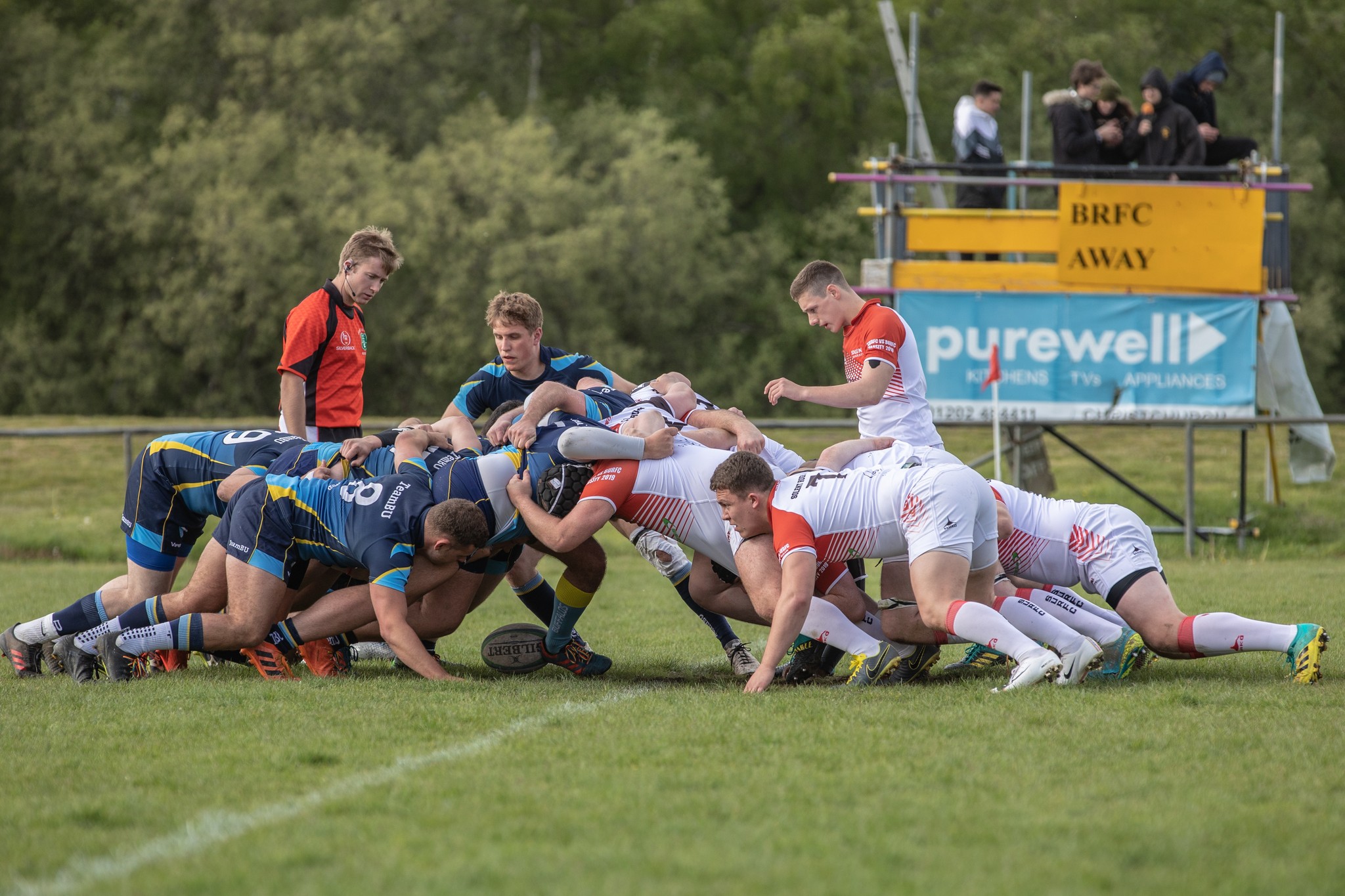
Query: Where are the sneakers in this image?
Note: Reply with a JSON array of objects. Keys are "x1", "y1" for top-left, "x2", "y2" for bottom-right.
[
  {"x1": 1278, "y1": 623, "x2": 1330, "y2": 686},
  {"x1": 41, "y1": 640, "x2": 69, "y2": 677},
  {"x1": 583, "y1": 641, "x2": 594, "y2": 653},
  {"x1": 350, "y1": 641, "x2": 396, "y2": 660},
  {"x1": 147, "y1": 650, "x2": 166, "y2": 675},
  {"x1": 726, "y1": 642, "x2": 760, "y2": 679},
  {"x1": 93, "y1": 629, "x2": 146, "y2": 684},
  {"x1": 154, "y1": 649, "x2": 192, "y2": 672},
  {"x1": 132, "y1": 653, "x2": 146, "y2": 679},
  {"x1": 541, "y1": 635, "x2": 612, "y2": 680},
  {"x1": 1056, "y1": 636, "x2": 1105, "y2": 686},
  {"x1": 239, "y1": 641, "x2": 302, "y2": 683},
  {"x1": 990, "y1": 649, "x2": 1064, "y2": 694},
  {"x1": 327, "y1": 634, "x2": 342, "y2": 678},
  {"x1": 942, "y1": 643, "x2": 1013, "y2": 674},
  {"x1": 193, "y1": 651, "x2": 227, "y2": 670},
  {"x1": 296, "y1": 637, "x2": 358, "y2": 679},
  {"x1": 889, "y1": 644, "x2": 941, "y2": 686},
  {"x1": 0, "y1": 622, "x2": 46, "y2": 678},
  {"x1": 846, "y1": 641, "x2": 902, "y2": 687},
  {"x1": 391, "y1": 652, "x2": 468, "y2": 671},
  {"x1": 786, "y1": 643, "x2": 822, "y2": 685},
  {"x1": 1088, "y1": 627, "x2": 1146, "y2": 681},
  {"x1": 51, "y1": 631, "x2": 104, "y2": 686}
]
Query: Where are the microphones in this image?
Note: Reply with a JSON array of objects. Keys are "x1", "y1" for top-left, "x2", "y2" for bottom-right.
[{"x1": 1141, "y1": 102, "x2": 1154, "y2": 120}]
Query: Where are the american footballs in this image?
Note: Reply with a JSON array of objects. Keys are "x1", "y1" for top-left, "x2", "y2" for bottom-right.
[{"x1": 476, "y1": 621, "x2": 550, "y2": 673}]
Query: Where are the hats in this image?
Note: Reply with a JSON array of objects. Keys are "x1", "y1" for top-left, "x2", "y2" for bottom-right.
[
  {"x1": 1206, "y1": 70, "x2": 1227, "y2": 84},
  {"x1": 1098, "y1": 78, "x2": 1123, "y2": 99}
]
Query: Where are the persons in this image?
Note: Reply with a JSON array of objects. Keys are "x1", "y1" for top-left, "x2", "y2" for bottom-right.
[
  {"x1": 0, "y1": 224, "x2": 1332, "y2": 694},
  {"x1": 950, "y1": 51, "x2": 1254, "y2": 259}
]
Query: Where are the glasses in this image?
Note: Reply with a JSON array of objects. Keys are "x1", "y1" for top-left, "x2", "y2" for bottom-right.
[{"x1": 1090, "y1": 82, "x2": 1104, "y2": 91}]
